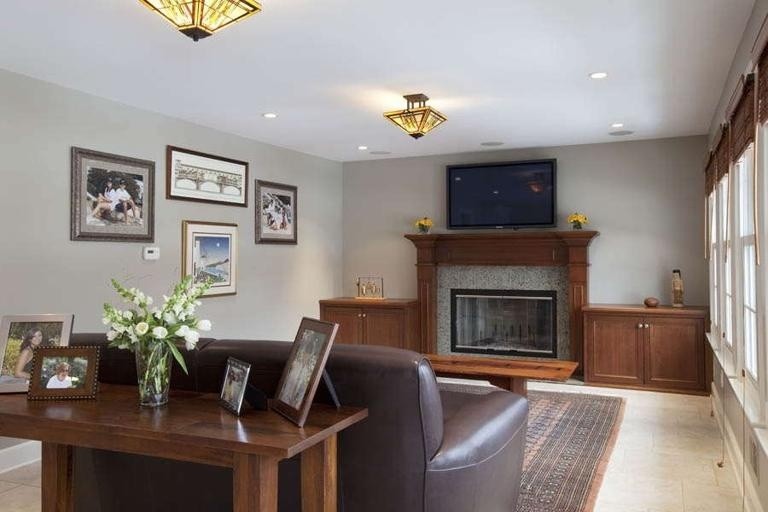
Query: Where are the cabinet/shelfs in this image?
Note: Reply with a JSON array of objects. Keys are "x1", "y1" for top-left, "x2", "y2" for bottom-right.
[
  {"x1": 581, "y1": 302, "x2": 711, "y2": 396},
  {"x1": 320, "y1": 296, "x2": 420, "y2": 353}
]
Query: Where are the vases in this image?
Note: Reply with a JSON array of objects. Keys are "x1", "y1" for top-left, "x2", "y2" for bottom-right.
[
  {"x1": 571, "y1": 223, "x2": 585, "y2": 231},
  {"x1": 419, "y1": 228, "x2": 432, "y2": 234},
  {"x1": 135, "y1": 342, "x2": 172, "y2": 408}
]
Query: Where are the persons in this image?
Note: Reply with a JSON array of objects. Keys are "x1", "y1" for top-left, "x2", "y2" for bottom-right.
[
  {"x1": 223, "y1": 370, "x2": 235, "y2": 402},
  {"x1": 46, "y1": 362, "x2": 73, "y2": 389},
  {"x1": 116, "y1": 179, "x2": 142, "y2": 226},
  {"x1": 280, "y1": 207, "x2": 288, "y2": 229},
  {"x1": 14, "y1": 328, "x2": 43, "y2": 384},
  {"x1": 90, "y1": 177, "x2": 116, "y2": 219},
  {"x1": 265, "y1": 199, "x2": 275, "y2": 226}
]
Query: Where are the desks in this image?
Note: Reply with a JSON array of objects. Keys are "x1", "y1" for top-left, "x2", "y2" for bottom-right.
[{"x1": 0, "y1": 384, "x2": 369, "y2": 512}]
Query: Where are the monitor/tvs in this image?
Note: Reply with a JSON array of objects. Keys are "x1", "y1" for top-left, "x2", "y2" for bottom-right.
[{"x1": 446, "y1": 158, "x2": 558, "y2": 228}]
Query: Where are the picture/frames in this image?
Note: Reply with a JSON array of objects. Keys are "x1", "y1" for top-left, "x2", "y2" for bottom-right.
[
  {"x1": 358, "y1": 278, "x2": 384, "y2": 297},
  {"x1": 69, "y1": 145, "x2": 297, "y2": 298},
  {"x1": 0, "y1": 313, "x2": 75, "y2": 393},
  {"x1": 27, "y1": 342, "x2": 101, "y2": 402},
  {"x1": 218, "y1": 356, "x2": 251, "y2": 416},
  {"x1": 271, "y1": 316, "x2": 340, "y2": 429}
]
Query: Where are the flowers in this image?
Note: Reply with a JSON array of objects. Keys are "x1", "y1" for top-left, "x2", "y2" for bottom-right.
[
  {"x1": 101, "y1": 275, "x2": 214, "y2": 396},
  {"x1": 415, "y1": 217, "x2": 433, "y2": 232},
  {"x1": 567, "y1": 213, "x2": 590, "y2": 228}
]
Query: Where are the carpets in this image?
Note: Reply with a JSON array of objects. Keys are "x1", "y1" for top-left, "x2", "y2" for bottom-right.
[{"x1": 436, "y1": 383, "x2": 626, "y2": 512}]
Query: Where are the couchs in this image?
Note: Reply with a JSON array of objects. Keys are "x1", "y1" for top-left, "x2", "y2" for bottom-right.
[{"x1": 69, "y1": 331, "x2": 529, "y2": 512}]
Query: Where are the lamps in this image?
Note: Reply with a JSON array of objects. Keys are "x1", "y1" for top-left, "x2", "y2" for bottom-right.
[
  {"x1": 142, "y1": 0, "x2": 262, "y2": 42},
  {"x1": 383, "y1": 93, "x2": 448, "y2": 140}
]
